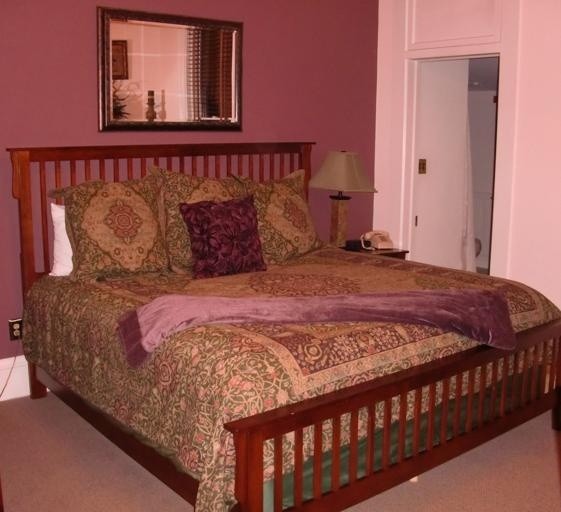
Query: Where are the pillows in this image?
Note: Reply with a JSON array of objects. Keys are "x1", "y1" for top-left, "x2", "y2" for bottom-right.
[{"x1": 46, "y1": 165, "x2": 344, "y2": 287}]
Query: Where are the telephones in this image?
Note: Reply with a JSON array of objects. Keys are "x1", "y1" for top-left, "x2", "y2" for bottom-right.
[{"x1": 363, "y1": 231, "x2": 394, "y2": 249}]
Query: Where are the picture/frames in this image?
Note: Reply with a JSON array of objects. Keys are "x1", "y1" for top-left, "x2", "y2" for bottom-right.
[{"x1": 112, "y1": 39, "x2": 130, "y2": 81}]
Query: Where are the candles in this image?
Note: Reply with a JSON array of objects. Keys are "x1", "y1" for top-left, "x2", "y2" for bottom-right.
[{"x1": 147, "y1": 90, "x2": 154, "y2": 104}]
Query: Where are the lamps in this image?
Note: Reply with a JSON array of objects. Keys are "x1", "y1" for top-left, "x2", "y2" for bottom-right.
[{"x1": 309, "y1": 149, "x2": 377, "y2": 248}]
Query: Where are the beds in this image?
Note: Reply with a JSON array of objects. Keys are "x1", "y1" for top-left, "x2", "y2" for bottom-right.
[{"x1": 5, "y1": 140, "x2": 561, "y2": 512}]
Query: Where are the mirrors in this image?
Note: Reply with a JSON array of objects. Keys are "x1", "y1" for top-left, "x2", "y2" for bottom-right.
[{"x1": 94, "y1": 5, "x2": 244, "y2": 132}]
